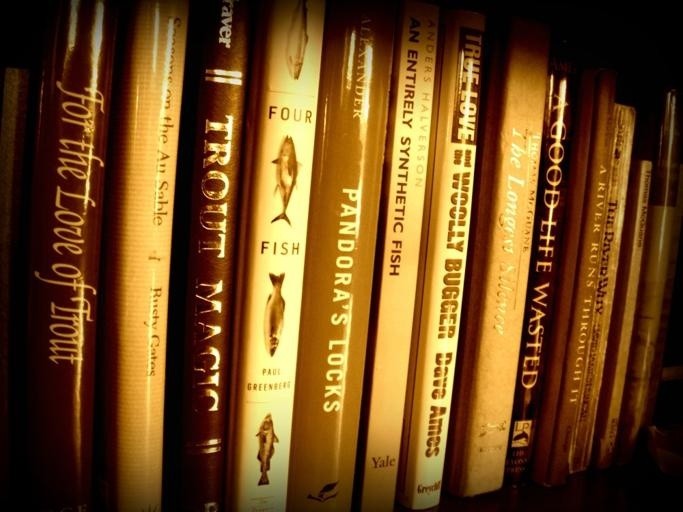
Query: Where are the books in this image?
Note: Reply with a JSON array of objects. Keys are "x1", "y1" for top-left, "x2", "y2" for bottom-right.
[{"x1": 0, "y1": 0, "x2": 682, "y2": 512}]
